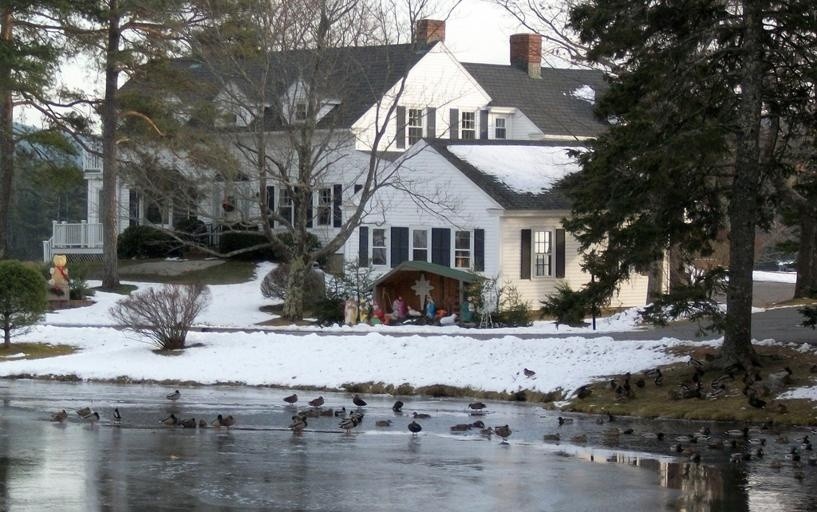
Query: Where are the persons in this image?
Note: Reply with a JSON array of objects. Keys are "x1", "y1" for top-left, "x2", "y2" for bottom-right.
[
  {"x1": 459, "y1": 296, "x2": 474, "y2": 322},
  {"x1": 391, "y1": 296, "x2": 400, "y2": 319},
  {"x1": 424, "y1": 295, "x2": 436, "y2": 324},
  {"x1": 397, "y1": 296, "x2": 406, "y2": 319},
  {"x1": 370, "y1": 314, "x2": 381, "y2": 326}
]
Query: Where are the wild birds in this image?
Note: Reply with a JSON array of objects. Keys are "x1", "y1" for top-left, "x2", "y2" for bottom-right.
[
  {"x1": 48, "y1": 406, "x2": 121, "y2": 424},
  {"x1": 165, "y1": 389, "x2": 181, "y2": 402},
  {"x1": 522, "y1": 367, "x2": 534, "y2": 376},
  {"x1": 542, "y1": 351, "x2": 817, "y2": 480},
  {"x1": 282, "y1": 392, "x2": 512, "y2": 446},
  {"x1": 158, "y1": 414, "x2": 234, "y2": 430}
]
